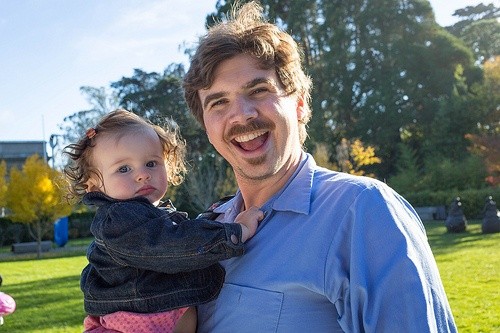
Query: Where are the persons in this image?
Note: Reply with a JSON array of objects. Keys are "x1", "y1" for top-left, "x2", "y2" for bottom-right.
[
  {"x1": 52, "y1": 106, "x2": 268, "y2": 333},
  {"x1": 183, "y1": 1, "x2": 457, "y2": 333}
]
